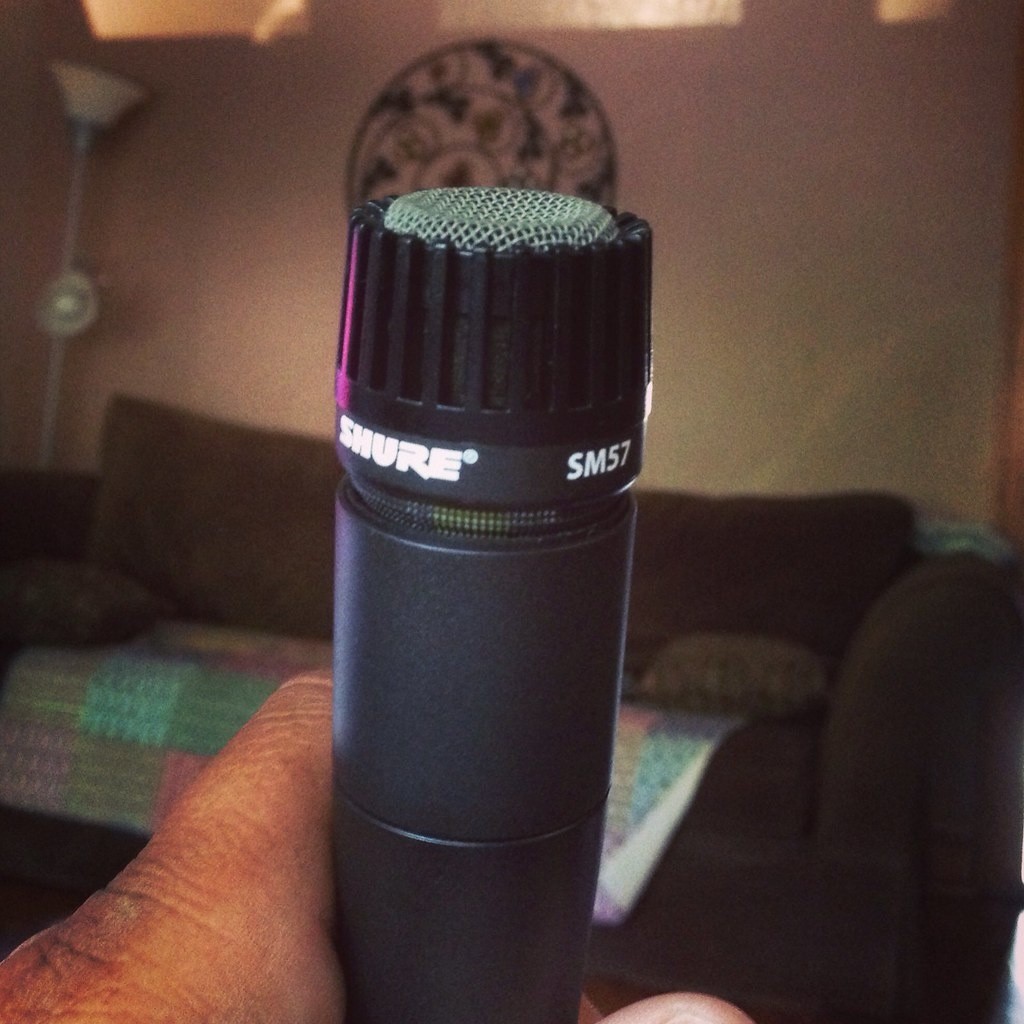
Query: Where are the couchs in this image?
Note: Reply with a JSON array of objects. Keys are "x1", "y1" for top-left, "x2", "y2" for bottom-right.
[{"x1": 1, "y1": 393, "x2": 1024, "y2": 1024}]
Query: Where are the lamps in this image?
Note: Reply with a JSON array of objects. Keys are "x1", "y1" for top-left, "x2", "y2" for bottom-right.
[{"x1": 23, "y1": 51, "x2": 154, "y2": 475}]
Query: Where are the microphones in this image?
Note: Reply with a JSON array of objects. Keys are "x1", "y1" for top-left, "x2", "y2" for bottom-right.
[{"x1": 326, "y1": 185, "x2": 656, "y2": 1024}]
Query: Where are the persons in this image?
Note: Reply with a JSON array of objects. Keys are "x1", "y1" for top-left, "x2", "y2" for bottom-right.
[{"x1": 0, "y1": 661, "x2": 759, "y2": 1024}]
